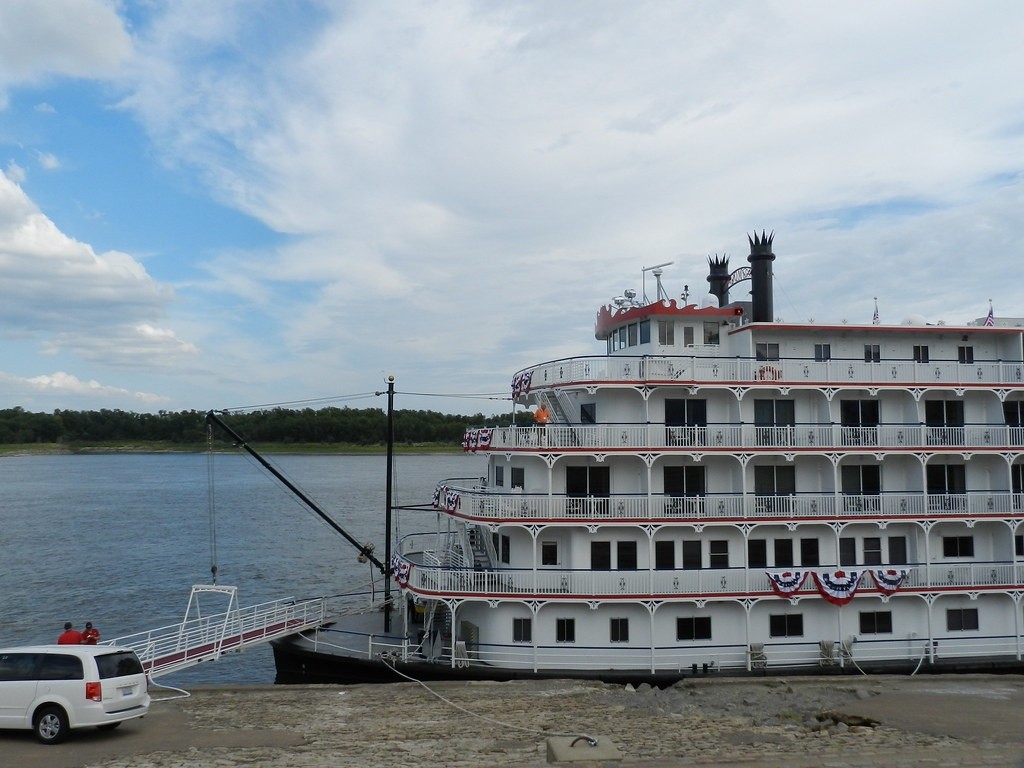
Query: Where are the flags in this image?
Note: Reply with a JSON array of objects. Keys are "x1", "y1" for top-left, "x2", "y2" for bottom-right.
[{"x1": 981, "y1": 309, "x2": 993, "y2": 326}]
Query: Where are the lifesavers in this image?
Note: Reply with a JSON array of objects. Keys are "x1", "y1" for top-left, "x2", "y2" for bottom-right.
[
  {"x1": 761, "y1": 368, "x2": 779, "y2": 381},
  {"x1": 736, "y1": 307, "x2": 744, "y2": 316}
]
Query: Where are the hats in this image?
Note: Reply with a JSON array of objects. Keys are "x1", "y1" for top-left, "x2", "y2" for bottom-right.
[{"x1": 86, "y1": 622, "x2": 92, "y2": 628}]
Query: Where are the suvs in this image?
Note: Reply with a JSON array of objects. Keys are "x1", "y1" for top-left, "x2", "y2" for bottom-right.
[{"x1": 0, "y1": 645, "x2": 151, "y2": 745}]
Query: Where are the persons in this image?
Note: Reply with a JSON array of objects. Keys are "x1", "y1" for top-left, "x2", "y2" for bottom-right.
[
  {"x1": 534, "y1": 403, "x2": 550, "y2": 436},
  {"x1": 56, "y1": 621, "x2": 100, "y2": 645}
]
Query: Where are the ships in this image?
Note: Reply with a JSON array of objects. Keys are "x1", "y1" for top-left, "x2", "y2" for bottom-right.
[{"x1": 207, "y1": 229, "x2": 1024, "y2": 691}]
